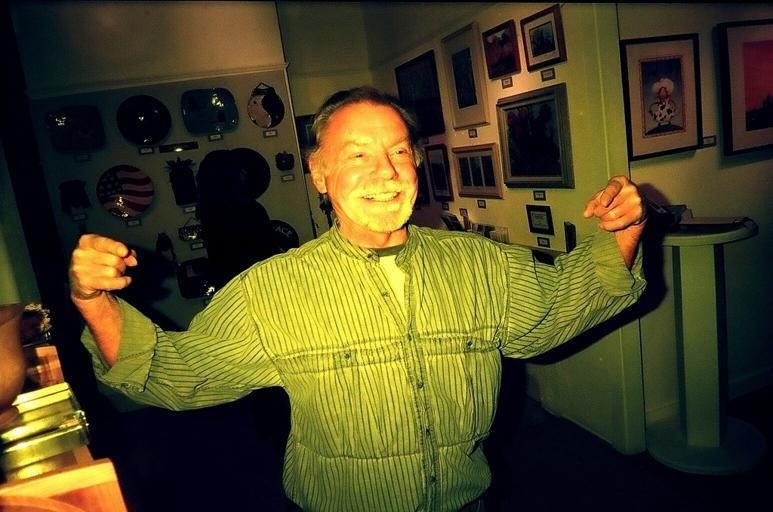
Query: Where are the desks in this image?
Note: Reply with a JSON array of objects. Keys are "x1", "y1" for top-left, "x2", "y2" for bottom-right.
[{"x1": 644, "y1": 218, "x2": 762, "y2": 473}]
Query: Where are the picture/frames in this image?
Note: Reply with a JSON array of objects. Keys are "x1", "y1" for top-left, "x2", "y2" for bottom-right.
[
  {"x1": 479, "y1": 18, "x2": 523, "y2": 81},
  {"x1": 523, "y1": 202, "x2": 556, "y2": 235},
  {"x1": 447, "y1": 141, "x2": 505, "y2": 204},
  {"x1": 295, "y1": 114, "x2": 318, "y2": 173},
  {"x1": 716, "y1": 18, "x2": 773, "y2": 156},
  {"x1": 420, "y1": 141, "x2": 459, "y2": 205},
  {"x1": 519, "y1": 4, "x2": 571, "y2": 73},
  {"x1": 392, "y1": 45, "x2": 449, "y2": 138},
  {"x1": 436, "y1": 19, "x2": 492, "y2": 131},
  {"x1": 490, "y1": 81, "x2": 574, "y2": 191},
  {"x1": 619, "y1": 32, "x2": 704, "y2": 162}
]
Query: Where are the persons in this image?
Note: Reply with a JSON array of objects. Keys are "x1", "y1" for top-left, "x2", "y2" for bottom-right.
[
  {"x1": 64, "y1": 83, "x2": 648, "y2": 510},
  {"x1": 491, "y1": 27, "x2": 515, "y2": 75},
  {"x1": 644, "y1": 86, "x2": 684, "y2": 136},
  {"x1": 485, "y1": 35, "x2": 505, "y2": 77}
]
